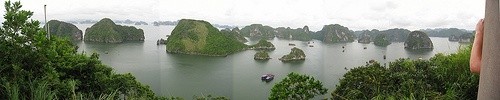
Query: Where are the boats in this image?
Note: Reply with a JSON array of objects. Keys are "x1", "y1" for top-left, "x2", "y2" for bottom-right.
[
  {"x1": 157, "y1": 40, "x2": 159, "y2": 45},
  {"x1": 261, "y1": 72, "x2": 274, "y2": 82},
  {"x1": 289, "y1": 44, "x2": 295, "y2": 46},
  {"x1": 159, "y1": 38, "x2": 164, "y2": 44}
]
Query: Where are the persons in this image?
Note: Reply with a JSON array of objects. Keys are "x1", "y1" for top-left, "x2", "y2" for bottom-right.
[{"x1": 468, "y1": 18, "x2": 485, "y2": 74}]
